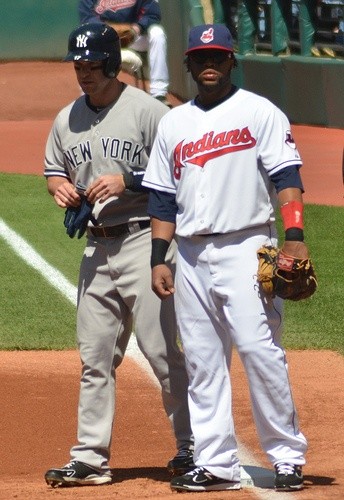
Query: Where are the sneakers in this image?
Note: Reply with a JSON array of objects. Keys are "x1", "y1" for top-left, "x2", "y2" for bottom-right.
[
  {"x1": 273, "y1": 461, "x2": 305, "y2": 491},
  {"x1": 44, "y1": 460, "x2": 113, "y2": 488},
  {"x1": 169, "y1": 465, "x2": 242, "y2": 492},
  {"x1": 166, "y1": 448, "x2": 197, "y2": 477}
]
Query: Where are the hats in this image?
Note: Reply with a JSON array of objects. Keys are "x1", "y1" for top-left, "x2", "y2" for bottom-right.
[{"x1": 183, "y1": 23, "x2": 236, "y2": 57}]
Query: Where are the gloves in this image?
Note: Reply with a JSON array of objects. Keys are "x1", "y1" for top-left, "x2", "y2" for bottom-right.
[{"x1": 63, "y1": 182, "x2": 99, "y2": 240}]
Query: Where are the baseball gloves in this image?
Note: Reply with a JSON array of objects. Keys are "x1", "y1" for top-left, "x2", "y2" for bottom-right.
[{"x1": 256, "y1": 244, "x2": 317, "y2": 301}]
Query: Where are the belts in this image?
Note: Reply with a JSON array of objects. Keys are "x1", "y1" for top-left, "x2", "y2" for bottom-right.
[{"x1": 88, "y1": 219, "x2": 152, "y2": 239}]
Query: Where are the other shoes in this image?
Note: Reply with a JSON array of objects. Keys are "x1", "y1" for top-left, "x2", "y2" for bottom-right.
[{"x1": 155, "y1": 95, "x2": 173, "y2": 108}]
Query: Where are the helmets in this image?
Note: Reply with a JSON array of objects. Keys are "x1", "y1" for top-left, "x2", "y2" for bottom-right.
[{"x1": 62, "y1": 22, "x2": 122, "y2": 79}]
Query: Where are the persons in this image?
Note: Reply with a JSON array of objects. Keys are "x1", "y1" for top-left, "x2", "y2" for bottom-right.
[
  {"x1": 77, "y1": 0, "x2": 174, "y2": 108},
  {"x1": 141, "y1": 25, "x2": 309, "y2": 490},
  {"x1": 43, "y1": 20, "x2": 196, "y2": 487}
]
